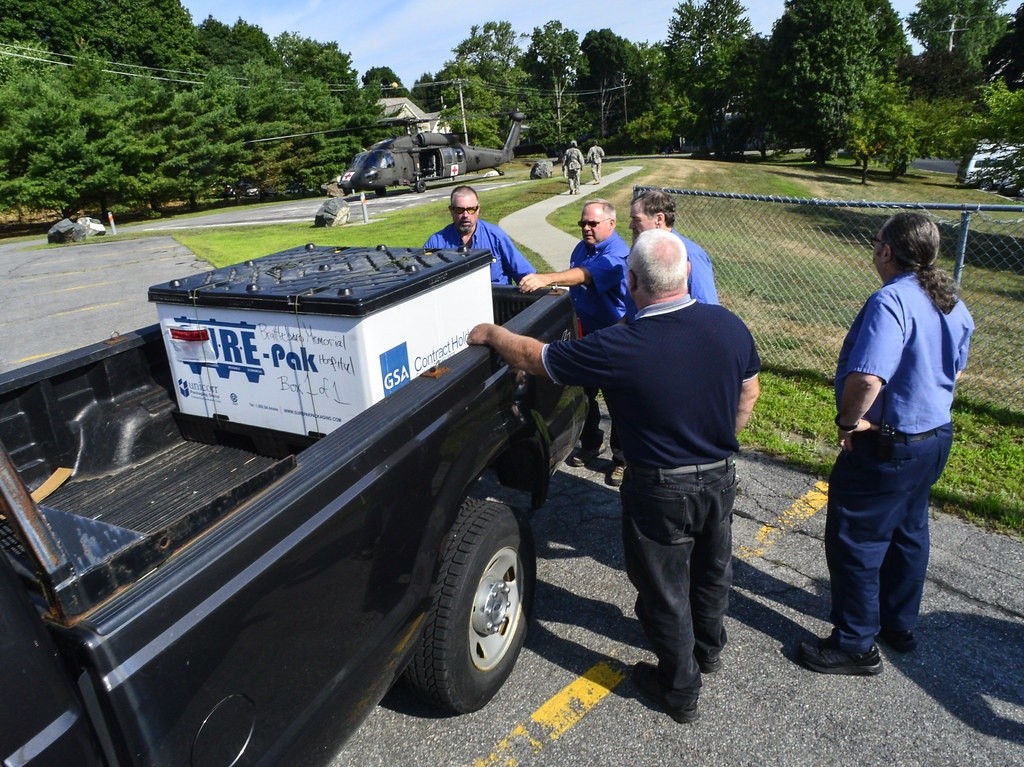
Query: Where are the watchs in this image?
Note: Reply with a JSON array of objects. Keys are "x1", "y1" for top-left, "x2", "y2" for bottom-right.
[{"x1": 834, "y1": 413, "x2": 859, "y2": 430}]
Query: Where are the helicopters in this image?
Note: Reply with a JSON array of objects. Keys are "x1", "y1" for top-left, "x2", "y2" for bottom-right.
[{"x1": 249, "y1": 104, "x2": 572, "y2": 196}]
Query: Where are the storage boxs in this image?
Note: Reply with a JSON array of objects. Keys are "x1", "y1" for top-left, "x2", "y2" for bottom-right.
[{"x1": 149, "y1": 243, "x2": 495, "y2": 458}]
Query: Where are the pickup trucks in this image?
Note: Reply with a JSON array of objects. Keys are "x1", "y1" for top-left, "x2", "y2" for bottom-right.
[{"x1": 0, "y1": 282, "x2": 591, "y2": 766}]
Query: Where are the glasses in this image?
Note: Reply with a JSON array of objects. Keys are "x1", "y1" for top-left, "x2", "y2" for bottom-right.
[
  {"x1": 577, "y1": 217, "x2": 611, "y2": 227},
  {"x1": 868, "y1": 234, "x2": 881, "y2": 247},
  {"x1": 450, "y1": 205, "x2": 479, "y2": 215}
]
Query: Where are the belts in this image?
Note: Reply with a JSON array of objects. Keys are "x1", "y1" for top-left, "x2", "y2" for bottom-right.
[
  {"x1": 630, "y1": 451, "x2": 736, "y2": 476},
  {"x1": 868, "y1": 425, "x2": 943, "y2": 444}
]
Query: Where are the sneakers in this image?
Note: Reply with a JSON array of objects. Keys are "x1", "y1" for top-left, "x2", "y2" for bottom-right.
[
  {"x1": 694, "y1": 652, "x2": 722, "y2": 674},
  {"x1": 878, "y1": 626, "x2": 917, "y2": 654},
  {"x1": 572, "y1": 440, "x2": 607, "y2": 467},
  {"x1": 631, "y1": 660, "x2": 701, "y2": 724},
  {"x1": 609, "y1": 465, "x2": 628, "y2": 486},
  {"x1": 796, "y1": 634, "x2": 885, "y2": 675}
]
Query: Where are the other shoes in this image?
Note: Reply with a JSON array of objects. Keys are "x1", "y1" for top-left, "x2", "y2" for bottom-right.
[
  {"x1": 574, "y1": 190, "x2": 581, "y2": 195},
  {"x1": 570, "y1": 190, "x2": 574, "y2": 195},
  {"x1": 593, "y1": 181, "x2": 600, "y2": 185}
]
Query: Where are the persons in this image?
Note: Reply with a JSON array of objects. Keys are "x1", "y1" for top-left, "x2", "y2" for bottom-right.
[
  {"x1": 424, "y1": 186, "x2": 537, "y2": 287},
  {"x1": 587, "y1": 140, "x2": 605, "y2": 184},
  {"x1": 615, "y1": 181, "x2": 720, "y2": 326},
  {"x1": 560, "y1": 140, "x2": 585, "y2": 195},
  {"x1": 467, "y1": 229, "x2": 761, "y2": 724},
  {"x1": 516, "y1": 196, "x2": 630, "y2": 484},
  {"x1": 795, "y1": 212, "x2": 977, "y2": 677}
]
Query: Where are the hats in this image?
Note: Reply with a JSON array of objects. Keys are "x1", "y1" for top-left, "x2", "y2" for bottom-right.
[
  {"x1": 570, "y1": 140, "x2": 577, "y2": 147},
  {"x1": 593, "y1": 140, "x2": 598, "y2": 146}
]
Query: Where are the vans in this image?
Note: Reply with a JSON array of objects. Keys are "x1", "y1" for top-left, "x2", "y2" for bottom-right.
[{"x1": 956, "y1": 142, "x2": 1024, "y2": 191}]
